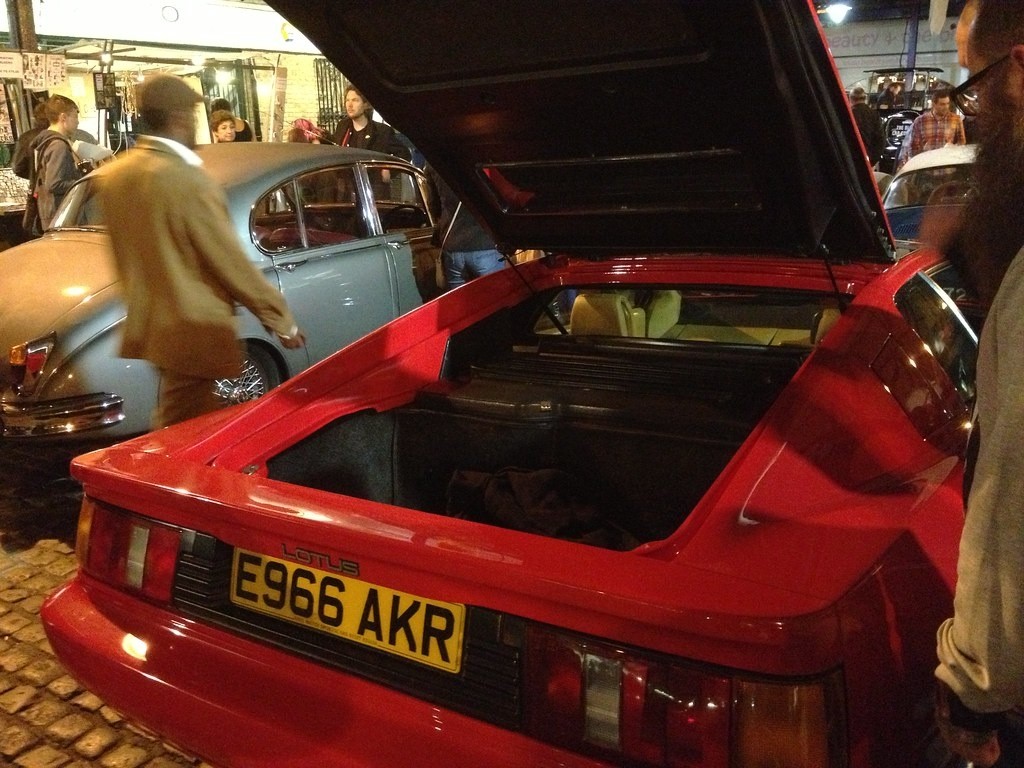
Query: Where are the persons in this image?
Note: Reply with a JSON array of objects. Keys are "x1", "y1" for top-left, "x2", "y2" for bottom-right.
[
  {"x1": 103, "y1": 74, "x2": 306, "y2": 428},
  {"x1": 11, "y1": 93, "x2": 93, "y2": 237},
  {"x1": 208, "y1": 84, "x2": 576, "y2": 324},
  {"x1": 849, "y1": 82, "x2": 966, "y2": 195},
  {"x1": 932, "y1": 0, "x2": 1024, "y2": 767}
]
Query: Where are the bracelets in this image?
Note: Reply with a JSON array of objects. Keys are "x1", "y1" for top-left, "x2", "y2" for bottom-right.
[{"x1": 941, "y1": 702, "x2": 1004, "y2": 744}]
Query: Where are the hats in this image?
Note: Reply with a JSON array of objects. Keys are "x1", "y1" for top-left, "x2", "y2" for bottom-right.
[{"x1": 142, "y1": 77, "x2": 207, "y2": 106}]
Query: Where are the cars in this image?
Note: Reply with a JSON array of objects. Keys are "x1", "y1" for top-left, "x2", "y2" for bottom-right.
[
  {"x1": 0, "y1": 139, "x2": 445, "y2": 486},
  {"x1": 31, "y1": 0, "x2": 1024, "y2": 768}
]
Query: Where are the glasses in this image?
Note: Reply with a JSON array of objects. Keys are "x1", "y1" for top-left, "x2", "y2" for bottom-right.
[{"x1": 949, "y1": 54, "x2": 1009, "y2": 116}]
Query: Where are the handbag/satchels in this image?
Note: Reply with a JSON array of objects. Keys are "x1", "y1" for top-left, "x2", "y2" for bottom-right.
[{"x1": 435, "y1": 258, "x2": 445, "y2": 287}]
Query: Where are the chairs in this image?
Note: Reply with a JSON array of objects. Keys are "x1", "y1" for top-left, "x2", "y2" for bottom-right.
[
  {"x1": 254, "y1": 225, "x2": 360, "y2": 252},
  {"x1": 571, "y1": 289, "x2": 842, "y2": 348}
]
